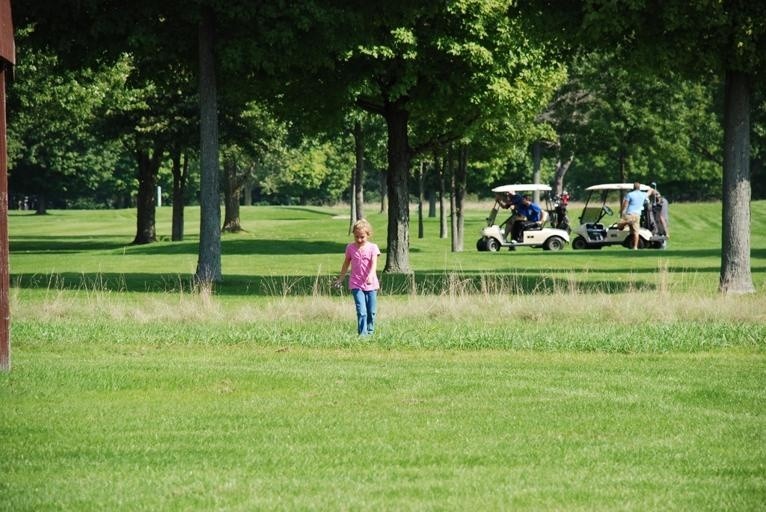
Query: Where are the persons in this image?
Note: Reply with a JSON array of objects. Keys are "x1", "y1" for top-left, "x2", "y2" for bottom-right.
[
  {"x1": 618, "y1": 182, "x2": 652, "y2": 250},
  {"x1": 648, "y1": 190, "x2": 670, "y2": 237},
  {"x1": 334, "y1": 218, "x2": 381, "y2": 335},
  {"x1": 495, "y1": 192, "x2": 521, "y2": 239},
  {"x1": 509, "y1": 195, "x2": 545, "y2": 250}
]
